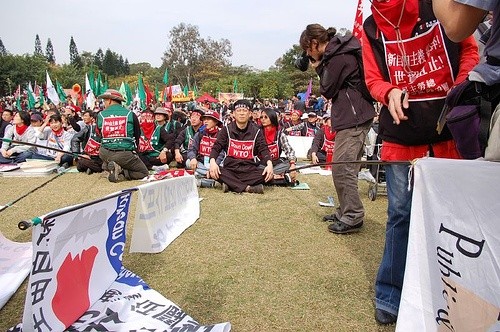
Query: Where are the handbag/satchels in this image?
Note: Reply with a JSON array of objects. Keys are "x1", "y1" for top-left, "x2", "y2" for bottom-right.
[{"x1": 444, "y1": 78, "x2": 492, "y2": 160}]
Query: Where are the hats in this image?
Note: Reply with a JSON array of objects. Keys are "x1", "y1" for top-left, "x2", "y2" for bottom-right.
[
  {"x1": 30, "y1": 114, "x2": 42, "y2": 121},
  {"x1": 308, "y1": 111, "x2": 317, "y2": 117},
  {"x1": 325, "y1": 114, "x2": 331, "y2": 123},
  {"x1": 154, "y1": 107, "x2": 169, "y2": 120},
  {"x1": 98, "y1": 89, "x2": 126, "y2": 102},
  {"x1": 200, "y1": 110, "x2": 223, "y2": 128}
]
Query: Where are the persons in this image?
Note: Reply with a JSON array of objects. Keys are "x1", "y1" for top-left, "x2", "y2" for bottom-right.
[
  {"x1": 0, "y1": 111, "x2": 36, "y2": 164},
  {"x1": 433, "y1": 0, "x2": 500, "y2": 161},
  {"x1": 36, "y1": 114, "x2": 72, "y2": 169},
  {"x1": 1, "y1": 92, "x2": 105, "y2": 134},
  {"x1": 252, "y1": 92, "x2": 333, "y2": 137},
  {"x1": 208, "y1": 99, "x2": 235, "y2": 124},
  {"x1": 207, "y1": 99, "x2": 274, "y2": 194},
  {"x1": 97, "y1": 91, "x2": 149, "y2": 183},
  {"x1": 186, "y1": 111, "x2": 226, "y2": 179},
  {"x1": 307, "y1": 117, "x2": 336, "y2": 170},
  {"x1": 174, "y1": 109, "x2": 205, "y2": 168},
  {"x1": 259, "y1": 108, "x2": 297, "y2": 182},
  {"x1": 138, "y1": 107, "x2": 174, "y2": 171},
  {"x1": 361, "y1": 0, "x2": 480, "y2": 323},
  {"x1": 71, "y1": 110, "x2": 103, "y2": 175},
  {"x1": 301, "y1": 24, "x2": 376, "y2": 233}
]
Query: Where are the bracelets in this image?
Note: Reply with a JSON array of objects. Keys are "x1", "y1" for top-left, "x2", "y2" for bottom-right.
[
  {"x1": 289, "y1": 162, "x2": 297, "y2": 165},
  {"x1": 289, "y1": 162, "x2": 296, "y2": 165}
]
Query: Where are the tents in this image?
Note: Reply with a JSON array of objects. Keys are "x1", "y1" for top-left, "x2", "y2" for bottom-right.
[{"x1": 195, "y1": 92, "x2": 220, "y2": 103}]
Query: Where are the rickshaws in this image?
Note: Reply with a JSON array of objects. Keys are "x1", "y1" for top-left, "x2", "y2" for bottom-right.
[{"x1": 368, "y1": 144, "x2": 388, "y2": 201}]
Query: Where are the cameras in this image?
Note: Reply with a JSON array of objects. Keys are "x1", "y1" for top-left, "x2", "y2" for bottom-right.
[{"x1": 294, "y1": 51, "x2": 315, "y2": 71}]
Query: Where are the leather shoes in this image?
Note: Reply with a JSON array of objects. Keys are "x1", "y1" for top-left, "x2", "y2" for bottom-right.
[{"x1": 376, "y1": 308, "x2": 396, "y2": 324}]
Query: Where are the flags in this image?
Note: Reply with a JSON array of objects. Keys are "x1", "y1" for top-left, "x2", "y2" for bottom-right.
[
  {"x1": 22, "y1": 191, "x2": 231, "y2": 332},
  {"x1": 0, "y1": 232, "x2": 32, "y2": 310},
  {"x1": 129, "y1": 175, "x2": 200, "y2": 253},
  {"x1": 395, "y1": 156, "x2": 500, "y2": 331},
  {"x1": 305, "y1": 78, "x2": 312, "y2": 100},
  {"x1": 83, "y1": 64, "x2": 198, "y2": 110},
  {"x1": 140, "y1": 168, "x2": 193, "y2": 182},
  {"x1": 14, "y1": 69, "x2": 67, "y2": 109}
]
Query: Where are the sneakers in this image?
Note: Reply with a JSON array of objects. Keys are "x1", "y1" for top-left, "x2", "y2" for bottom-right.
[
  {"x1": 108, "y1": 160, "x2": 121, "y2": 182},
  {"x1": 323, "y1": 214, "x2": 337, "y2": 221},
  {"x1": 327, "y1": 220, "x2": 363, "y2": 233}
]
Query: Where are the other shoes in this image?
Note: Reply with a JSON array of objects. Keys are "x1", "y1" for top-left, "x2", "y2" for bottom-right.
[
  {"x1": 86, "y1": 168, "x2": 92, "y2": 174},
  {"x1": 156, "y1": 164, "x2": 169, "y2": 172},
  {"x1": 248, "y1": 184, "x2": 264, "y2": 193},
  {"x1": 222, "y1": 183, "x2": 228, "y2": 192}
]
